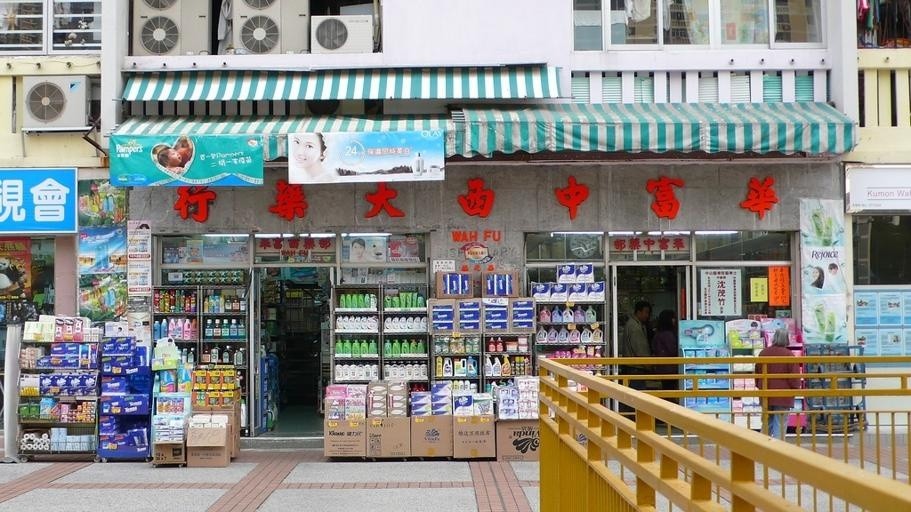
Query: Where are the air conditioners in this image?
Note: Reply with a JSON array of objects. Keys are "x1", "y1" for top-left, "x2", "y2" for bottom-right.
[
  {"x1": 18, "y1": 76, "x2": 93, "y2": 131},
  {"x1": 130, "y1": 1, "x2": 375, "y2": 117}
]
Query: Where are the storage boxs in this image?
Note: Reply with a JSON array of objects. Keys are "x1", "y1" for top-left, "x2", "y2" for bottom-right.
[
  {"x1": 425, "y1": 298, "x2": 482, "y2": 333},
  {"x1": 152, "y1": 400, "x2": 241, "y2": 467},
  {"x1": 478, "y1": 298, "x2": 537, "y2": 334},
  {"x1": 435, "y1": 271, "x2": 474, "y2": 299},
  {"x1": 481, "y1": 271, "x2": 519, "y2": 298},
  {"x1": 323, "y1": 414, "x2": 540, "y2": 462}
]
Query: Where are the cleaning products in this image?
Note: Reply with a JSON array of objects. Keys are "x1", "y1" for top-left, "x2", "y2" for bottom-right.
[{"x1": 554, "y1": 345, "x2": 603, "y2": 370}]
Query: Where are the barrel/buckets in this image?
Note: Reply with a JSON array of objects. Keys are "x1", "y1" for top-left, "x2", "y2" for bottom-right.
[{"x1": 535, "y1": 305, "x2": 604, "y2": 345}]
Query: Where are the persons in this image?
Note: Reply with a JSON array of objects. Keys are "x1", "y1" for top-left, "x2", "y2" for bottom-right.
[
  {"x1": 622, "y1": 300, "x2": 655, "y2": 412},
  {"x1": 647, "y1": 308, "x2": 679, "y2": 391},
  {"x1": 350, "y1": 238, "x2": 372, "y2": 263},
  {"x1": 752, "y1": 330, "x2": 803, "y2": 444},
  {"x1": 293, "y1": 132, "x2": 341, "y2": 184},
  {"x1": 827, "y1": 262, "x2": 839, "y2": 276},
  {"x1": 808, "y1": 266, "x2": 825, "y2": 289},
  {"x1": 153, "y1": 136, "x2": 194, "y2": 175}
]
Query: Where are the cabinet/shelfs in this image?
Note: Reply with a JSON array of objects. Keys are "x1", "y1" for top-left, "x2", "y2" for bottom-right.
[
  {"x1": 531, "y1": 284, "x2": 606, "y2": 373},
  {"x1": 154, "y1": 284, "x2": 258, "y2": 435},
  {"x1": 326, "y1": 285, "x2": 432, "y2": 393},
  {"x1": 678, "y1": 343, "x2": 870, "y2": 432},
  {"x1": 432, "y1": 335, "x2": 533, "y2": 393},
  {"x1": 0, "y1": 323, "x2": 151, "y2": 462}
]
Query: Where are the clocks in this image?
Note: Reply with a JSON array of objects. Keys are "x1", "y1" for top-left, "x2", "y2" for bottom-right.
[{"x1": 569, "y1": 235, "x2": 598, "y2": 259}]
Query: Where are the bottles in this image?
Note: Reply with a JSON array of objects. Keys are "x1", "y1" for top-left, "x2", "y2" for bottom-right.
[
  {"x1": 152, "y1": 283, "x2": 246, "y2": 368},
  {"x1": 453, "y1": 379, "x2": 479, "y2": 393},
  {"x1": 335, "y1": 361, "x2": 379, "y2": 380},
  {"x1": 435, "y1": 335, "x2": 503, "y2": 354},
  {"x1": 337, "y1": 293, "x2": 429, "y2": 331}
]
